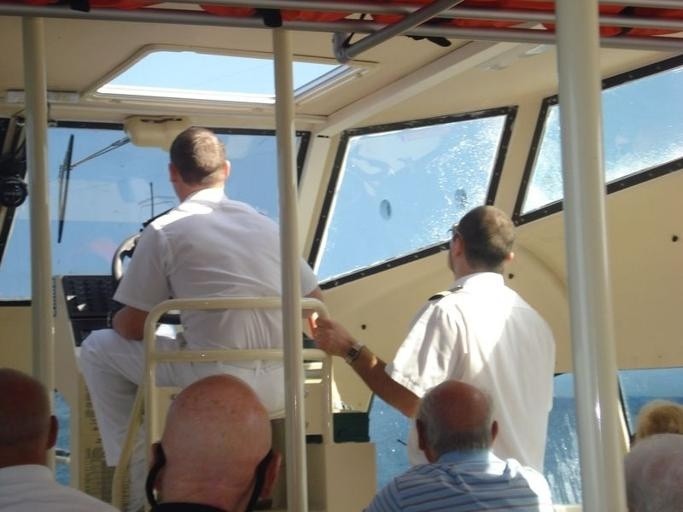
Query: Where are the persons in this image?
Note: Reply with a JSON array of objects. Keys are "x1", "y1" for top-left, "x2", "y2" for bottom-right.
[
  {"x1": 624, "y1": 433, "x2": 683, "y2": 512},
  {"x1": 311, "y1": 203, "x2": 558, "y2": 475},
  {"x1": 358, "y1": 379, "x2": 545, "y2": 512},
  {"x1": 77, "y1": 126, "x2": 326, "y2": 512},
  {"x1": 0, "y1": 366, "x2": 122, "y2": 512},
  {"x1": 144, "y1": 372, "x2": 283, "y2": 512},
  {"x1": 633, "y1": 399, "x2": 683, "y2": 440}
]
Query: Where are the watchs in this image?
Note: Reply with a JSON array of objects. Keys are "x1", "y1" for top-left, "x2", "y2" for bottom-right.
[{"x1": 344, "y1": 341, "x2": 365, "y2": 366}]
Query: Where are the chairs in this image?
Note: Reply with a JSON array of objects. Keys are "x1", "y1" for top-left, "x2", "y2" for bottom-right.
[{"x1": 110, "y1": 292, "x2": 339, "y2": 512}]
